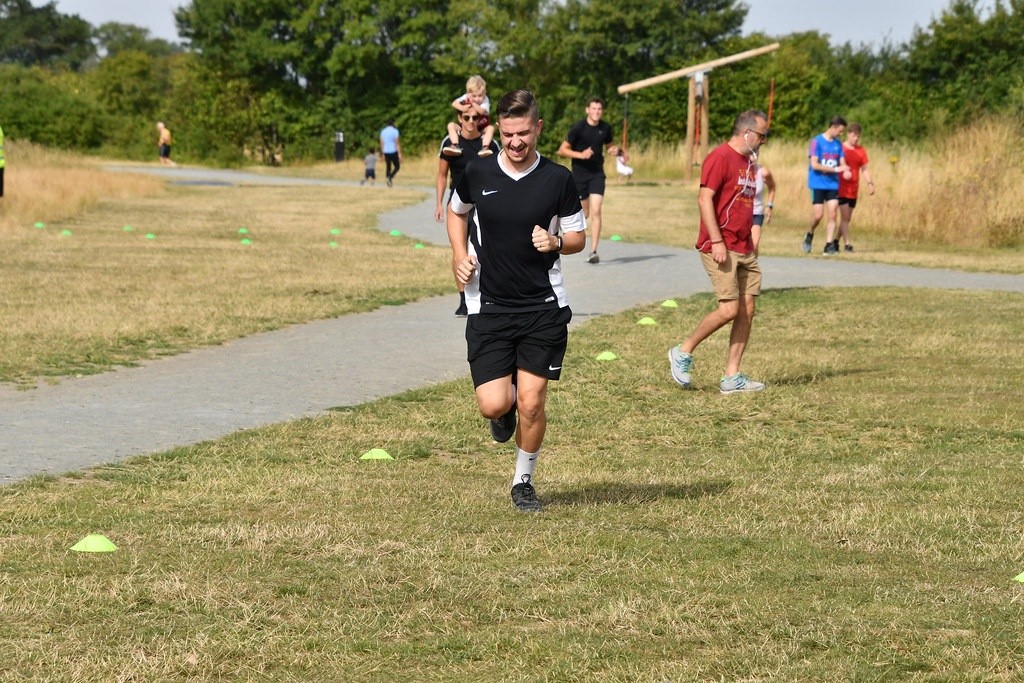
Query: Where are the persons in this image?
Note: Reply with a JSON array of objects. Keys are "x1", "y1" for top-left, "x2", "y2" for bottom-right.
[
  {"x1": 380, "y1": 118, "x2": 401, "y2": 185},
  {"x1": 446, "y1": 88, "x2": 588, "y2": 507},
  {"x1": 559, "y1": 96, "x2": 618, "y2": 263},
  {"x1": 803, "y1": 115, "x2": 852, "y2": 256},
  {"x1": 834, "y1": 124, "x2": 874, "y2": 253},
  {"x1": 616, "y1": 148, "x2": 633, "y2": 182},
  {"x1": 157, "y1": 122, "x2": 173, "y2": 164},
  {"x1": 669, "y1": 106, "x2": 767, "y2": 394},
  {"x1": 749, "y1": 151, "x2": 775, "y2": 258},
  {"x1": 443, "y1": 75, "x2": 495, "y2": 156},
  {"x1": 360, "y1": 148, "x2": 377, "y2": 186},
  {"x1": 436, "y1": 105, "x2": 501, "y2": 317}
]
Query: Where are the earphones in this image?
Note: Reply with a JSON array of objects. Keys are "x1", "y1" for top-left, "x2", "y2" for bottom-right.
[{"x1": 744, "y1": 134, "x2": 749, "y2": 141}]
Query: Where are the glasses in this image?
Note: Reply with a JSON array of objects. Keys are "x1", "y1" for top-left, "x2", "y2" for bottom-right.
[
  {"x1": 462, "y1": 115, "x2": 480, "y2": 122},
  {"x1": 746, "y1": 130, "x2": 769, "y2": 141}
]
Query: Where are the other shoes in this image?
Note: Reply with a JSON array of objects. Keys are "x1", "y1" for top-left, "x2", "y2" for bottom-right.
[
  {"x1": 833, "y1": 240, "x2": 840, "y2": 250},
  {"x1": 454, "y1": 301, "x2": 468, "y2": 319},
  {"x1": 802, "y1": 232, "x2": 813, "y2": 252},
  {"x1": 823, "y1": 243, "x2": 837, "y2": 256},
  {"x1": 478, "y1": 147, "x2": 493, "y2": 157},
  {"x1": 443, "y1": 144, "x2": 462, "y2": 156},
  {"x1": 845, "y1": 245, "x2": 853, "y2": 250},
  {"x1": 586, "y1": 252, "x2": 600, "y2": 264}
]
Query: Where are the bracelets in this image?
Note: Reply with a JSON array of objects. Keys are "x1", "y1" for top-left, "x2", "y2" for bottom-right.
[
  {"x1": 868, "y1": 182, "x2": 873, "y2": 185},
  {"x1": 767, "y1": 205, "x2": 772, "y2": 210},
  {"x1": 835, "y1": 167, "x2": 837, "y2": 173},
  {"x1": 711, "y1": 240, "x2": 723, "y2": 244}
]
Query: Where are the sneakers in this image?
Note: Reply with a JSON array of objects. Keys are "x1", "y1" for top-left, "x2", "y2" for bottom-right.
[
  {"x1": 720, "y1": 373, "x2": 764, "y2": 394},
  {"x1": 490, "y1": 382, "x2": 518, "y2": 443},
  {"x1": 511, "y1": 474, "x2": 543, "y2": 514},
  {"x1": 668, "y1": 344, "x2": 693, "y2": 386}
]
